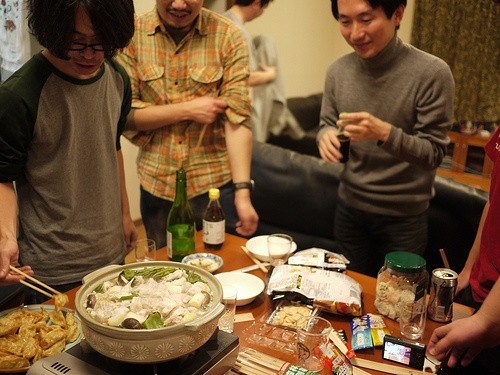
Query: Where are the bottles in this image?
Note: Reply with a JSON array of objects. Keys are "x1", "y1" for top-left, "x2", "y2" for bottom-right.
[
  {"x1": 201, "y1": 188, "x2": 226, "y2": 250},
  {"x1": 335, "y1": 112, "x2": 351, "y2": 164},
  {"x1": 374, "y1": 251, "x2": 429, "y2": 323},
  {"x1": 166, "y1": 169, "x2": 196, "y2": 261}
]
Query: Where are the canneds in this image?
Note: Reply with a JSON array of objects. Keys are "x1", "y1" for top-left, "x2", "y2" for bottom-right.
[{"x1": 426, "y1": 268, "x2": 458, "y2": 323}]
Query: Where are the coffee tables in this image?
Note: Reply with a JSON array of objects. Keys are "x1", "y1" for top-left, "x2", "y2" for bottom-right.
[{"x1": 435, "y1": 130, "x2": 493, "y2": 193}]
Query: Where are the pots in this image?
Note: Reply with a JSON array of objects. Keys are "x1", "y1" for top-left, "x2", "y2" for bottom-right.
[{"x1": 75, "y1": 260, "x2": 223, "y2": 362}]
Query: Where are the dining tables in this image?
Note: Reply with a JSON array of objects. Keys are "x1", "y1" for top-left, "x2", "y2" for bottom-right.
[{"x1": 42, "y1": 230, "x2": 475, "y2": 375}]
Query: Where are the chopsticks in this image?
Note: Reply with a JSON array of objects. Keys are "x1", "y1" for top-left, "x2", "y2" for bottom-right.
[
  {"x1": 232, "y1": 262, "x2": 270, "y2": 273},
  {"x1": 240, "y1": 245, "x2": 268, "y2": 273},
  {"x1": 7, "y1": 264, "x2": 60, "y2": 299}
]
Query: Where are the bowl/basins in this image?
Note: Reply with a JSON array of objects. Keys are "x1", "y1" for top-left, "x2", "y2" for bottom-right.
[
  {"x1": 215, "y1": 272, "x2": 265, "y2": 306},
  {"x1": 246, "y1": 236, "x2": 298, "y2": 262}
]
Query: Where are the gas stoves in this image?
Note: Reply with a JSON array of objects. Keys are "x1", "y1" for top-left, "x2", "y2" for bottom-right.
[{"x1": 26, "y1": 326, "x2": 240, "y2": 375}]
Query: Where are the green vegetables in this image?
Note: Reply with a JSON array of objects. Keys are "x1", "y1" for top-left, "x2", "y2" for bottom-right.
[{"x1": 93, "y1": 267, "x2": 208, "y2": 331}]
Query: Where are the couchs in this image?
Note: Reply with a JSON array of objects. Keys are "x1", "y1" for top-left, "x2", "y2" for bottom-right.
[
  {"x1": 253, "y1": 142, "x2": 487, "y2": 292},
  {"x1": 266, "y1": 92, "x2": 350, "y2": 163}
]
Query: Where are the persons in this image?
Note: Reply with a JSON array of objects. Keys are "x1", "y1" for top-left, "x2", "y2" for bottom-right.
[
  {"x1": 112, "y1": 0, "x2": 258, "y2": 252},
  {"x1": 426, "y1": 125, "x2": 500, "y2": 375},
  {"x1": 316, "y1": 0, "x2": 455, "y2": 278},
  {"x1": 0, "y1": 0, "x2": 137, "y2": 304},
  {"x1": 221, "y1": 0, "x2": 305, "y2": 151}
]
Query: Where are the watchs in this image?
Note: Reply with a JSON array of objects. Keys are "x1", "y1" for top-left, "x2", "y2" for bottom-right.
[{"x1": 233, "y1": 179, "x2": 255, "y2": 191}]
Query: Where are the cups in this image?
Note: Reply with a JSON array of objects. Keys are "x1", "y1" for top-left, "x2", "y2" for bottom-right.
[
  {"x1": 135, "y1": 239, "x2": 156, "y2": 262},
  {"x1": 218, "y1": 285, "x2": 238, "y2": 334},
  {"x1": 400, "y1": 302, "x2": 426, "y2": 340},
  {"x1": 268, "y1": 233, "x2": 293, "y2": 268},
  {"x1": 295, "y1": 316, "x2": 332, "y2": 373}
]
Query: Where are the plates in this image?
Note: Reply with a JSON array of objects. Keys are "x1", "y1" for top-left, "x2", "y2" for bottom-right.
[
  {"x1": 182, "y1": 252, "x2": 223, "y2": 273},
  {"x1": 0, "y1": 304, "x2": 85, "y2": 371}
]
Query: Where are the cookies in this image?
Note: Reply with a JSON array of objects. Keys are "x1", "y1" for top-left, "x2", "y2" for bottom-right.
[{"x1": 272, "y1": 300, "x2": 311, "y2": 330}]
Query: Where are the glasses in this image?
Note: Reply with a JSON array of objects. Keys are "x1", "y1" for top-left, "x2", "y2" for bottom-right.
[{"x1": 69, "y1": 42, "x2": 117, "y2": 51}]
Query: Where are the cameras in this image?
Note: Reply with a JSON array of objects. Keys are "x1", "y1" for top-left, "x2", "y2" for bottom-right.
[{"x1": 381, "y1": 334, "x2": 427, "y2": 370}]
